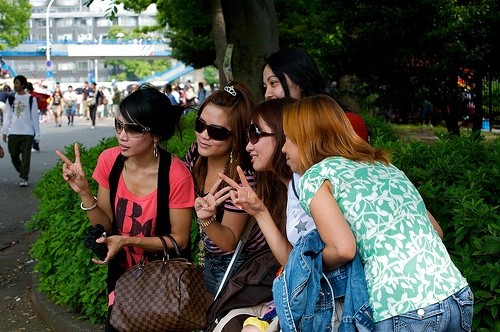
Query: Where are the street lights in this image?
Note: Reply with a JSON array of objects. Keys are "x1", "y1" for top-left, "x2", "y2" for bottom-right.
[{"x1": 94, "y1": 33, "x2": 125, "y2": 82}]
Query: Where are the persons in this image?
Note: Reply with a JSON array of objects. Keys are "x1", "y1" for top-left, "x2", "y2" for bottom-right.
[
  {"x1": 63, "y1": 32, "x2": 171, "y2": 44},
  {"x1": 3, "y1": 75, "x2": 40, "y2": 188},
  {"x1": 47, "y1": 80, "x2": 215, "y2": 130},
  {"x1": 56, "y1": 83, "x2": 194, "y2": 332},
  {"x1": 0, "y1": 81, "x2": 39, "y2": 158},
  {"x1": 207, "y1": 48, "x2": 474, "y2": 332},
  {"x1": 182, "y1": 81, "x2": 275, "y2": 310}
]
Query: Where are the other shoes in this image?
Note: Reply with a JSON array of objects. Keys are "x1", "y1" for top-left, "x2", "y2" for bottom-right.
[
  {"x1": 92, "y1": 126, "x2": 95, "y2": 130},
  {"x1": 20, "y1": 179, "x2": 29, "y2": 187}
]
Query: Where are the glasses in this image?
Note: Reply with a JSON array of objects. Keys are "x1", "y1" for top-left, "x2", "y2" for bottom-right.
[
  {"x1": 246, "y1": 123, "x2": 275, "y2": 145},
  {"x1": 195, "y1": 117, "x2": 232, "y2": 140},
  {"x1": 115, "y1": 117, "x2": 150, "y2": 138}
]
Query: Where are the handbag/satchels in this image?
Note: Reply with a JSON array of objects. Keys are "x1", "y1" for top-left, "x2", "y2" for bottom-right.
[
  {"x1": 86, "y1": 98, "x2": 97, "y2": 107},
  {"x1": 110, "y1": 234, "x2": 208, "y2": 332}
]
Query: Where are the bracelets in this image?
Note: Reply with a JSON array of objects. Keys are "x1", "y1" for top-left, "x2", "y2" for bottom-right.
[
  {"x1": 80, "y1": 196, "x2": 98, "y2": 210},
  {"x1": 198, "y1": 214, "x2": 217, "y2": 228}
]
